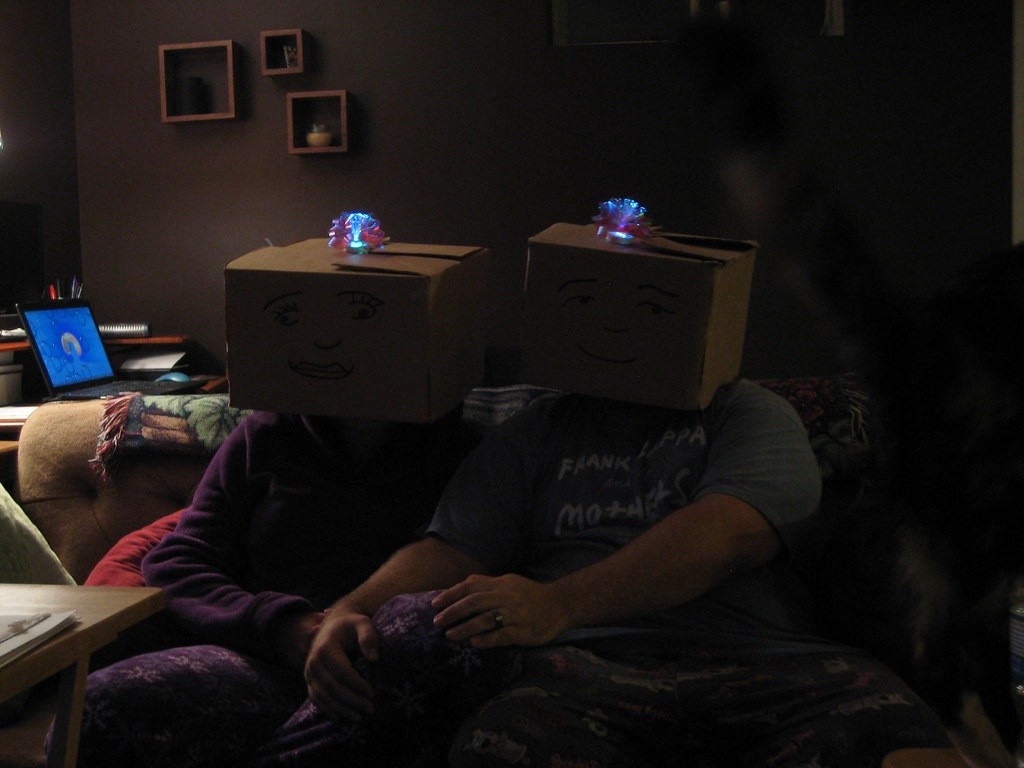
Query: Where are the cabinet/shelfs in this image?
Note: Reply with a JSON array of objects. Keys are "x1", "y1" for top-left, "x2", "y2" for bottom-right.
[{"x1": 157, "y1": 28, "x2": 351, "y2": 156}]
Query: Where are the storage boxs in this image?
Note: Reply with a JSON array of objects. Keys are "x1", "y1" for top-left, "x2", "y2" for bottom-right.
[
  {"x1": 515, "y1": 221, "x2": 763, "y2": 414},
  {"x1": 221, "y1": 236, "x2": 498, "y2": 431}
]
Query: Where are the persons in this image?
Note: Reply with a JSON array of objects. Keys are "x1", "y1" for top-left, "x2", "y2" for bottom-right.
[
  {"x1": 44, "y1": 232, "x2": 511, "y2": 768},
  {"x1": 300, "y1": 200, "x2": 949, "y2": 768}
]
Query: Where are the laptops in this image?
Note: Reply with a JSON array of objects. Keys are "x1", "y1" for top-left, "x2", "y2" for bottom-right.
[{"x1": 14, "y1": 295, "x2": 213, "y2": 402}]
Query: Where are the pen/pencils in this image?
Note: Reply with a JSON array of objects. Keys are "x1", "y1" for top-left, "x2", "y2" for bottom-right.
[{"x1": 49, "y1": 271, "x2": 87, "y2": 300}]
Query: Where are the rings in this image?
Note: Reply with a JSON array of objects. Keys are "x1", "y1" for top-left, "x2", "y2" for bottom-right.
[{"x1": 488, "y1": 608, "x2": 506, "y2": 629}]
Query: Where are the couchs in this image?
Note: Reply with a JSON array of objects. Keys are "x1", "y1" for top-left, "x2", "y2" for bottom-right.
[{"x1": 15, "y1": 377, "x2": 1024, "y2": 768}]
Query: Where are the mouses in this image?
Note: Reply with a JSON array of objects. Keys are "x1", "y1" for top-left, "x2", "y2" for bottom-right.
[{"x1": 155, "y1": 372, "x2": 191, "y2": 385}]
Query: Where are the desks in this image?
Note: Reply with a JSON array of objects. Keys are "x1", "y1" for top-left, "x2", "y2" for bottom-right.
[{"x1": 0, "y1": 581, "x2": 166, "y2": 768}]
